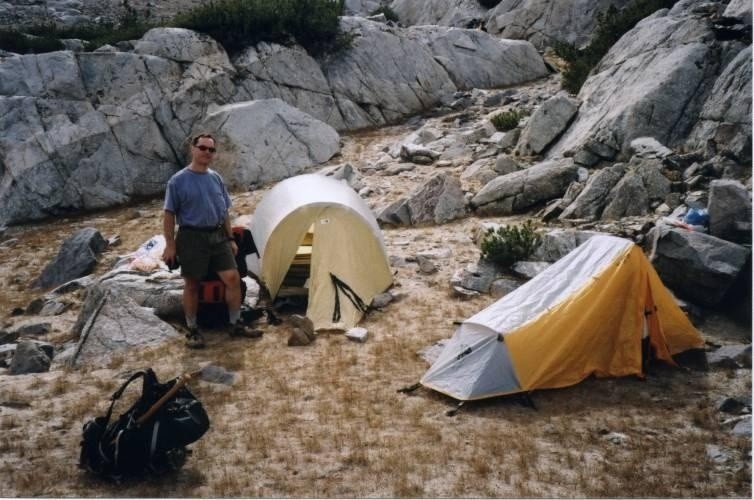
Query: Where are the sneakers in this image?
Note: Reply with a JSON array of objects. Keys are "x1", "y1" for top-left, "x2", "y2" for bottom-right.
[
  {"x1": 185, "y1": 328, "x2": 204, "y2": 350},
  {"x1": 228, "y1": 319, "x2": 264, "y2": 337}
]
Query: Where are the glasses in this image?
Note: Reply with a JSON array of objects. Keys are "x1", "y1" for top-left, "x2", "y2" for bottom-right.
[{"x1": 194, "y1": 144, "x2": 216, "y2": 154}]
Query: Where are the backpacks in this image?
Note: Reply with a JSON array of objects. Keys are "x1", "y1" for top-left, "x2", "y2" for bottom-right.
[{"x1": 78, "y1": 363, "x2": 212, "y2": 485}]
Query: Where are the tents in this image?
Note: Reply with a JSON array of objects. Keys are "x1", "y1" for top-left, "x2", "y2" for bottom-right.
[
  {"x1": 417, "y1": 233, "x2": 707, "y2": 407},
  {"x1": 246, "y1": 172, "x2": 395, "y2": 334}
]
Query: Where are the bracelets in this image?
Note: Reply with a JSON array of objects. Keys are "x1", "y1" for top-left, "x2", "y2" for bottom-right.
[{"x1": 226, "y1": 235, "x2": 236, "y2": 242}]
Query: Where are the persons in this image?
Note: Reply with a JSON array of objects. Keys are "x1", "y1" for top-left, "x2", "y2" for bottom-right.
[{"x1": 160, "y1": 134, "x2": 264, "y2": 350}]
